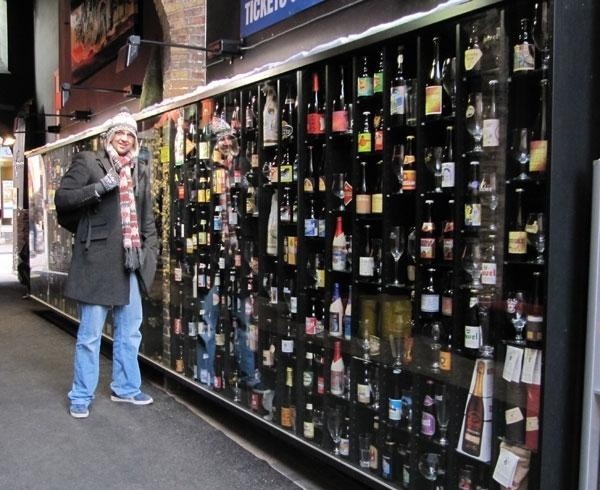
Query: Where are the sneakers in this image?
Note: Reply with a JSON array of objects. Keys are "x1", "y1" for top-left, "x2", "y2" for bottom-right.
[
  {"x1": 111, "y1": 392, "x2": 153, "y2": 405},
  {"x1": 69, "y1": 403, "x2": 89, "y2": 417}
]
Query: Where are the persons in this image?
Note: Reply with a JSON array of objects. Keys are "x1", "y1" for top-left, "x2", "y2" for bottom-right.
[
  {"x1": 51, "y1": 104, "x2": 162, "y2": 420},
  {"x1": 193, "y1": 115, "x2": 273, "y2": 398}
]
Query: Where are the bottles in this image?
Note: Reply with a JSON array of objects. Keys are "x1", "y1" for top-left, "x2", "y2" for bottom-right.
[
  {"x1": 461, "y1": 358, "x2": 486, "y2": 455},
  {"x1": 172, "y1": 1, "x2": 550, "y2": 490}
]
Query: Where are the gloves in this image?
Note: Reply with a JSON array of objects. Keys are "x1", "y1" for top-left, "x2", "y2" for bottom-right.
[{"x1": 100, "y1": 168, "x2": 120, "y2": 191}]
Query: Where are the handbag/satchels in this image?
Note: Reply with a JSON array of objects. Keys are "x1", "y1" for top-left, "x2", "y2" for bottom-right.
[{"x1": 56, "y1": 205, "x2": 80, "y2": 233}]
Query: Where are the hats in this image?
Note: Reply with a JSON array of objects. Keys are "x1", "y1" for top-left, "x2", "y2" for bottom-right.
[{"x1": 106, "y1": 107, "x2": 139, "y2": 156}]
